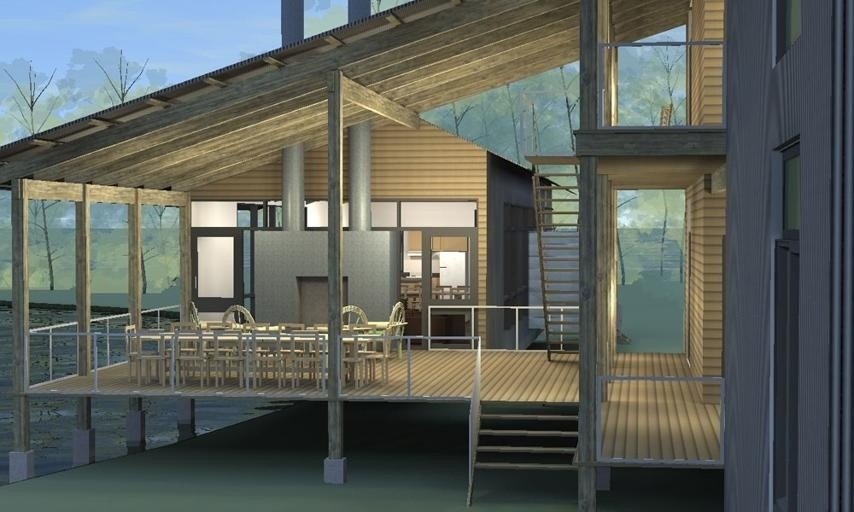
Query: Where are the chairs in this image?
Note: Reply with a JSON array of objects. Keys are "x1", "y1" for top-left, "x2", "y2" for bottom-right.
[{"x1": 124, "y1": 301, "x2": 408, "y2": 391}]
